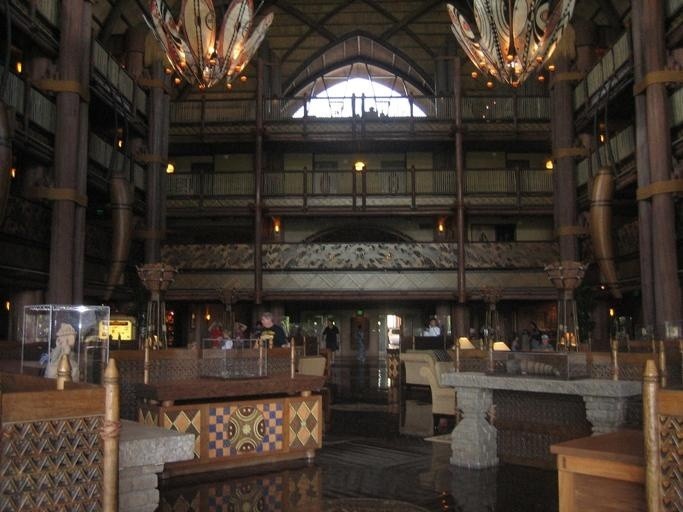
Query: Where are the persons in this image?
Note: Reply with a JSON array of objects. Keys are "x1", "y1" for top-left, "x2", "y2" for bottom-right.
[
  {"x1": 352, "y1": 323, "x2": 368, "y2": 364},
  {"x1": 42, "y1": 321, "x2": 80, "y2": 383},
  {"x1": 207, "y1": 320, "x2": 224, "y2": 351},
  {"x1": 420, "y1": 318, "x2": 440, "y2": 337},
  {"x1": 232, "y1": 322, "x2": 247, "y2": 349},
  {"x1": 321, "y1": 318, "x2": 339, "y2": 367},
  {"x1": 539, "y1": 334, "x2": 555, "y2": 352},
  {"x1": 251, "y1": 312, "x2": 287, "y2": 350},
  {"x1": 509, "y1": 335, "x2": 521, "y2": 352}
]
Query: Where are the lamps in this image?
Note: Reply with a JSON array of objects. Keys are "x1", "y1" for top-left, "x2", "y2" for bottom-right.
[
  {"x1": 140, "y1": 0, "x2": 276, "y2": 93},
  {"x1": 445, "y1": 0, "x2": 578, "y2": 90}
]
